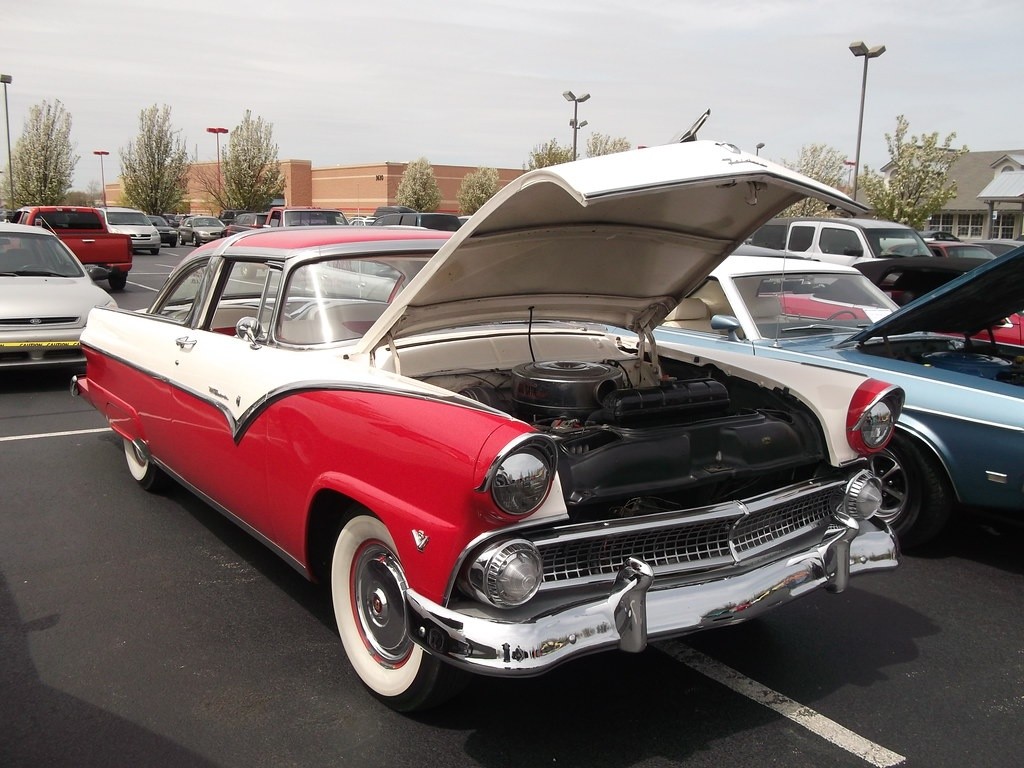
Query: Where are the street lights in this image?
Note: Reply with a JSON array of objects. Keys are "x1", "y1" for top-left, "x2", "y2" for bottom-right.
[
  {"x1": 848, "y1": 41, "x2": 887, "y2": 202},
  {"x1": 562, "y1": 90, "x2": 591, "y2": 161},
  {"x1": 206, "y1": 127, "x2": 228, "y2": 220},
  {"x1": 0, "y1": 74, "x2": 16, "y2": 222},
  {"x1": 93, "y1": 150, "x2": 110, "y2": 208}
]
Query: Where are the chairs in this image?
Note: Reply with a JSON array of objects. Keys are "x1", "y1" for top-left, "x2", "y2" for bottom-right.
[
  {"x1": 660, "y1": 297, "x2": 720, "y2": 334},
  {"x1": 4, "y1": 248, "x2": 33, "y2": 272},
  {"x1": 42, "y1": 217, "x2": 56, "y2": 229},
  {"x1": 745, "y1": 296, "x2": 786, "y2": 324},
  {"x1": 68, "y1": 217, "x2": 79, "y2": 228}
]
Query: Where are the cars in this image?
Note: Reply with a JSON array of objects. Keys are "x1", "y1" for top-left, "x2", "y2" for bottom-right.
[
  {"x1": 145, "y1": 214, "x2": 178, "y2": 248},
  {"x1": 160, "y1": 205, "x2": 474, "y2": 247},
  {"x1": 657, "y1": 214, "x2": 1024, "y2": 552},
  {"x1": 93, "y1": 206, "x2": 161, "y2": 255},
  {"x1": 64, "y1": 143, "x2": 908, "y2": 718},
  {"x1": 0, "y1": 222, "x2": 121, "y2": 386}
]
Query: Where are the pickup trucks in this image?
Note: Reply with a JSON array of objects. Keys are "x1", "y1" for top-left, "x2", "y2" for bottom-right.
[
  {"x1": 224, "y1": 205, "x2": 351, "y2": 238},
  {"x1": 10, "y1": 205, "x2": 134, "y2": 291}
]
